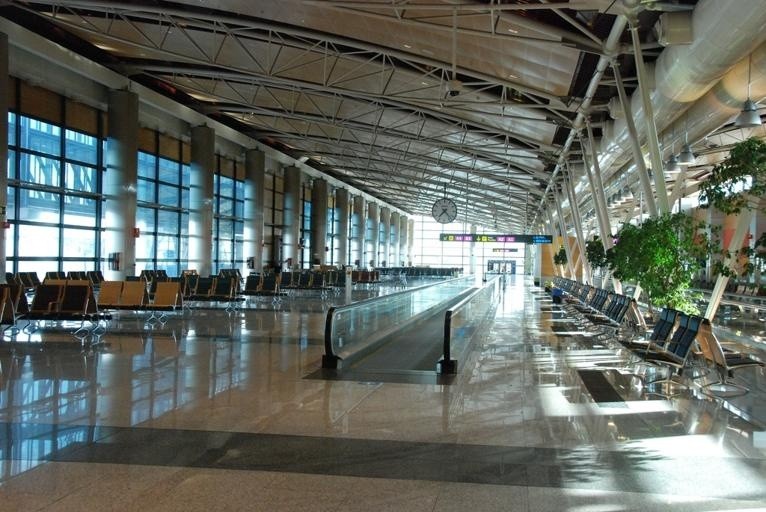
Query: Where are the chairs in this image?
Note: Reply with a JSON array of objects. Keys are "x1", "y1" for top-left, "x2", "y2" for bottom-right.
[{"x1": 543, "y1": 273, "x2": 766, "y2": 399}]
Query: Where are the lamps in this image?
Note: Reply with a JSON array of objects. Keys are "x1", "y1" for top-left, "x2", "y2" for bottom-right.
[
  {"x1": 606, "y1": 109, "x2": 696, "y2": 210},
  {"x1": 731, "y1": 46, "x2": 762, "y2": 128}
]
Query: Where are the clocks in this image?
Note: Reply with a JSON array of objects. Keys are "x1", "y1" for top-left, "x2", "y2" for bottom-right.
[{"x1": 431, "y1": 198, "x2": 457, "y2": 224}]
